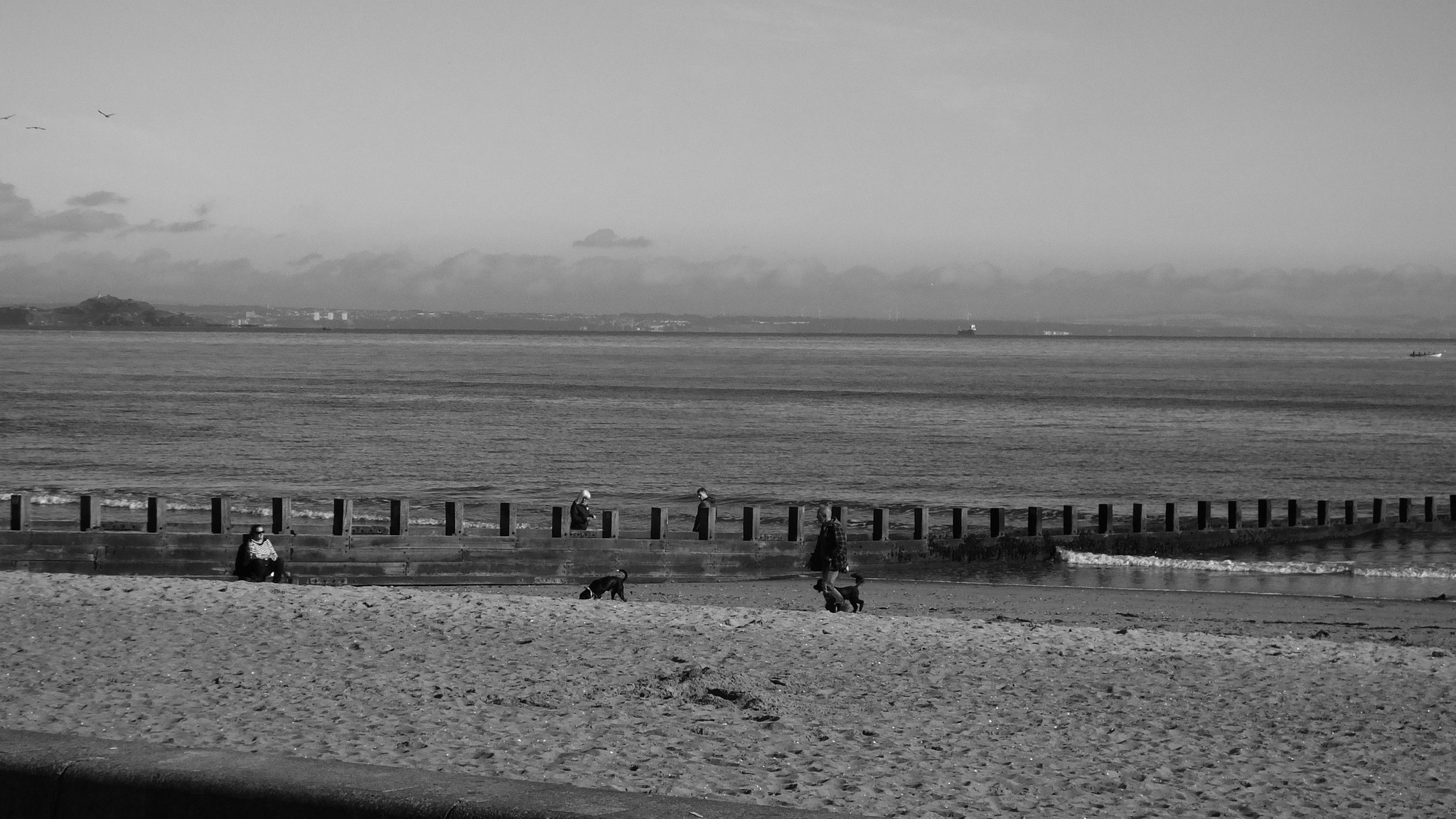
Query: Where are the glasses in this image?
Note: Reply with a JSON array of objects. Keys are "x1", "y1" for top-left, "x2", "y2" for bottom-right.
[{"x1": 254, "y1": 531, "x2": 265, "y2": 534}]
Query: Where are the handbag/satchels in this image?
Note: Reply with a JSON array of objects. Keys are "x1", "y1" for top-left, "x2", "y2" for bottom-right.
[{"x1": 833, "y1": 556, "x2": 848, "y2": 573}]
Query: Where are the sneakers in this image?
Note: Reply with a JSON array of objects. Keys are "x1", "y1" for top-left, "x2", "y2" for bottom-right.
[{"x1": 837, "y1": 600, "x2": 850, "y2": 612}]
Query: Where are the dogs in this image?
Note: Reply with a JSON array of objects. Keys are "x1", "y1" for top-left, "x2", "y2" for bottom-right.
[
  {"x1": 579, "y1": 569, "x2": 629, "y2": 601},
  {"x1": 813, "y1": 573, "x2": 864, "y2": 614}
]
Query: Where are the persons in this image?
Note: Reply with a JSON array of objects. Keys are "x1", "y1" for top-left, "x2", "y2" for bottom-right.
[
  {"x1": 807, "y1": 506, "x2": 850, "y2": 612},
  {"x1": 693, "y1": 488, "x2": 717, "y2": 532},
  {"x1": 244, "y1": 524, "x2": 284, "y2": 583},
  {"x1": 570, "y1": 490, "x2": 596, "y2": 530}
]
